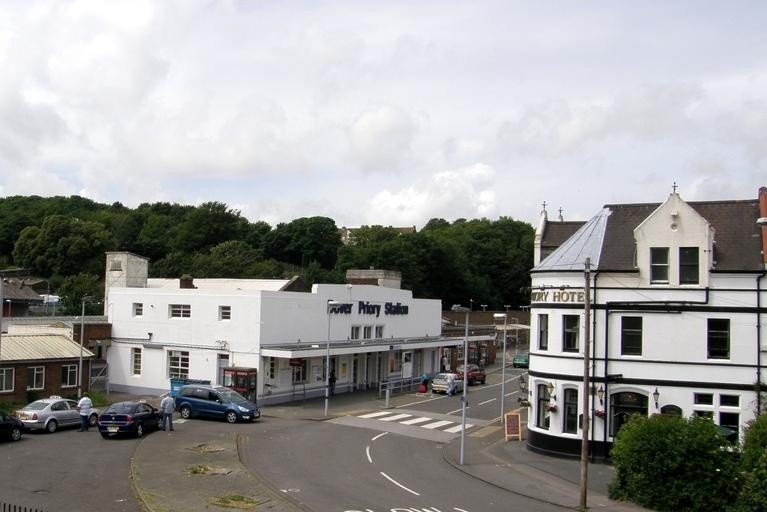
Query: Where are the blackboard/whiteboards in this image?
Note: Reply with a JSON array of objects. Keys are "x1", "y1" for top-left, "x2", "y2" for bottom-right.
[{"x1": 504, "y1": 413, "x2": 521, "y2": 436}]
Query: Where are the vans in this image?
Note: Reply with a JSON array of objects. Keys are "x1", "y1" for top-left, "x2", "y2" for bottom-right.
[{"x1": 174, "y1": 383, "x2": 260, "y2": 423}]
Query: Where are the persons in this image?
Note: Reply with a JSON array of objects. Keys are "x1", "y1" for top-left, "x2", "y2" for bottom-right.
[
  {"x1": 328, "y1": 369, "x2": 336, "y2": 395},
  {"x1": 443, "y1": 370, "x2": 454, "y2": 396},
  {"x1": 441, "y1": 355, "x2": 447, "y2": 372},
  {"x1": 160, "y1": 393, "x2": 176, "y2": 431},
  {"x1": 76, "y1": 392, "x2": 92, "y2": 431}
]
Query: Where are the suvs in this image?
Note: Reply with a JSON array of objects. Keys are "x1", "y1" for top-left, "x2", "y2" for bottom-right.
[{"x1": 455, "y1": 364, "x2": 486, "y2": 385}]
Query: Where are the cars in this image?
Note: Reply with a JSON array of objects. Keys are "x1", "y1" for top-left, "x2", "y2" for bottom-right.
[
  {"x1": 512, "y1": 353, "x2": 528, "y2": 368},
  {"x1": 0, "y1": 411, "x2": 24, "y2": 442},
  {"x1": 15, "y1": 395, "x2": 103, "y2": 433},
  {"x1": 431, "y1": 373, "x2": 465, "y2": 394},
  {"x1": 97, "y1": 399, "x2": 163, "y2": 439}
]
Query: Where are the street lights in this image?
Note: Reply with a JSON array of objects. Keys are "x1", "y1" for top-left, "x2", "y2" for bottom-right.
[
  {"x1": 517, "y1": 306, "x2": 530, "y2": 312},
  {"x1": 469, "y1": 299, "x2": 473, "y2": 311},
  {"x1": 450, "y1": 304, "x2": 469, "y2": 466},
  {"x1": 511, "y1": 317, "x2": 518, "y2": 358},
  {"x1": 480, "y1": 304, "x2": 488, "y2": 312},
  {"x1": 491, "y1": 312, "x2": 507, "y2": 424},
  {"x1": 77, "y1": 294, "x2": 93, "y2": 402},
  {"x1": 323, "y1": 301, "x2": 343, "y2": 417},
  {"x1": 5, "y1": 299, "x2": 11, "y2": 316},
  {"x1": 503, "y1": 304, "x2": 511, "y2": 311}
]
